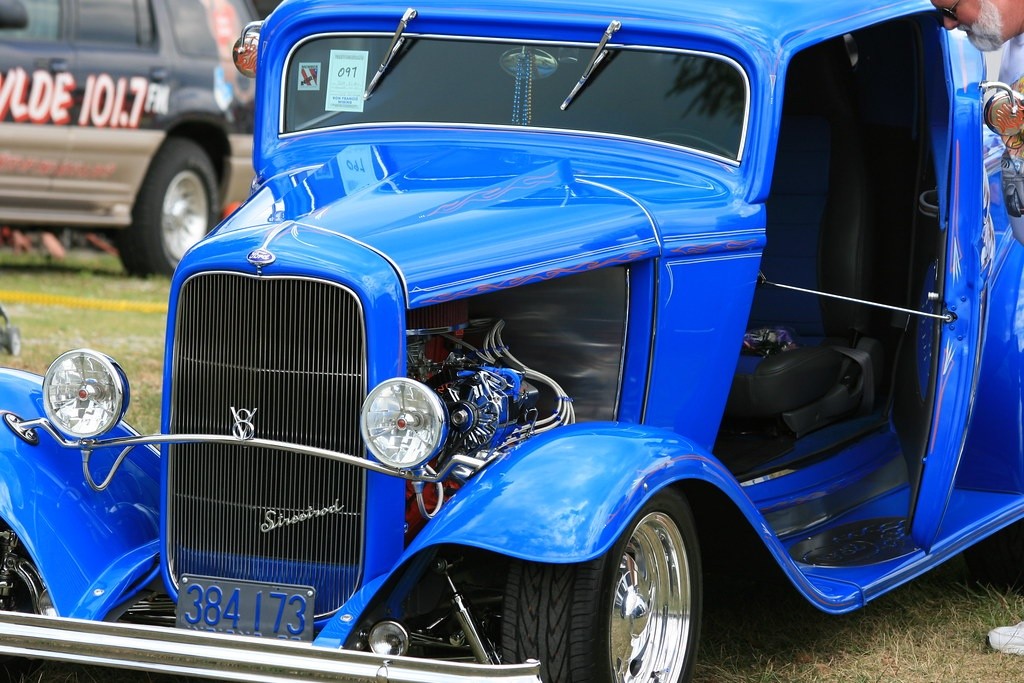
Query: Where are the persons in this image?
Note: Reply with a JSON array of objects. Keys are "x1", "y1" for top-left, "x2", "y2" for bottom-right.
[{"x1": 932, "y1": 0, "x2": 1024, "y2": 657}]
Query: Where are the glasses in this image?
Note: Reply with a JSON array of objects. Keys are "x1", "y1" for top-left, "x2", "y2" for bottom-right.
[{"x1": 939, "y1": 0, "x2": 966, "y2": 21}]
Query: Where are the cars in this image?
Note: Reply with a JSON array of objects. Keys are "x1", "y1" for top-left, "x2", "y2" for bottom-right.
[
  {"x1": 0, "y1": 0, "x2": 1024, "y2": 683},
  {"x1": 0, "y1": 0, "x2": 277, "y2": 282}
]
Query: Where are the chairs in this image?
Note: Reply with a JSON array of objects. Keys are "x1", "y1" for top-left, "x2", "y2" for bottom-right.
[{"x1": 726, "y1": 105, "x2": 885, "y2": 441}]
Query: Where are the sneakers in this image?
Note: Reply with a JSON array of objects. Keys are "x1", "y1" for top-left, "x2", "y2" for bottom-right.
[{"x1": 989, "y1": 622, "x2": 1020, "y2": 654}]
